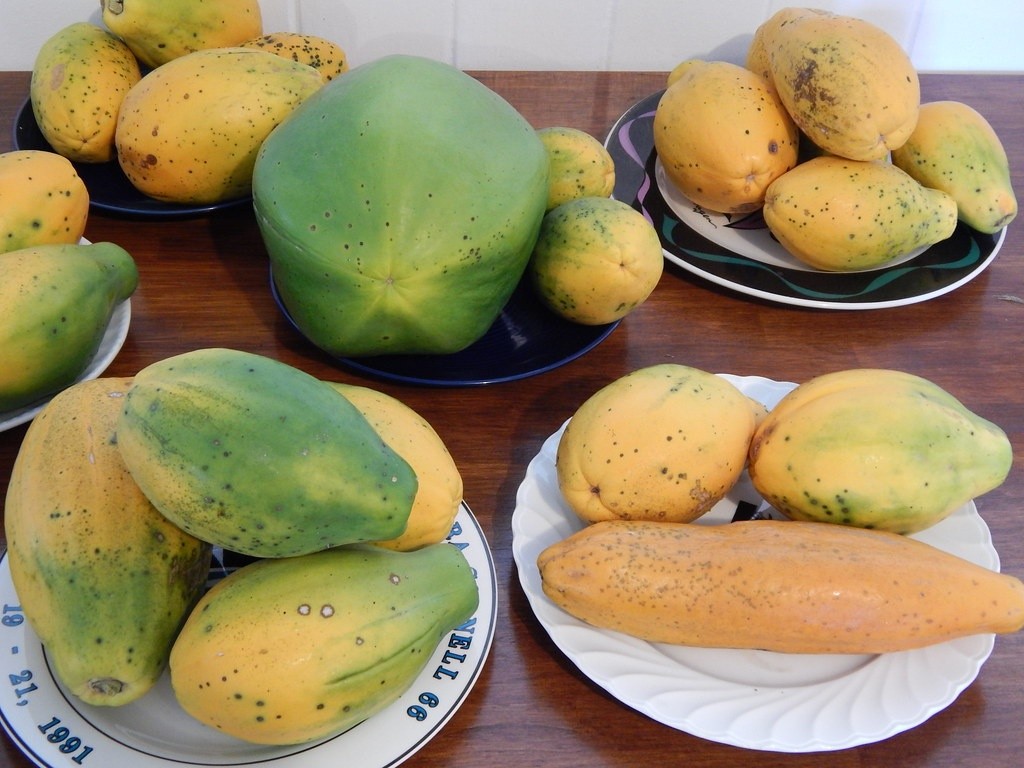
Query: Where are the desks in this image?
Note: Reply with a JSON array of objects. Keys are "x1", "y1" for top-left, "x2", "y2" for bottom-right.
[{"x1": 0, "y1": 69, "x2": 1024, "y2": 768}]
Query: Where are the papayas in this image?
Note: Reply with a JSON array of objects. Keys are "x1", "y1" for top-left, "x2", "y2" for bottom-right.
[
  {"x1": 0, "y1": 148, "x2": 140, "y2": 409},
  {"x1": 253, "y1": 53, "x2": 664, "y2": 359},
  {"x1": 6, "y1": 347, "x2": 480, "y2": 748},
  {"x1": 534, "y1": 362, "x2": 1024, "y2": 654},
  {"x1": 29, "y1": 0, "x2": 350, "y2": 206},
  {"x1": 651, "y1": 6, "x2": 1017, "y2": 271}
]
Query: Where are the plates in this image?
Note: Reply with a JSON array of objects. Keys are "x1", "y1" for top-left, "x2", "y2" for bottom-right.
[
  {"x1": 270, "y1": 266, "x2": 623, "y2": 386},
  {"x1": 14, "y1": 94, "x2": 254, "y2": 215},
  {"x1": 0, "y1": 500, "x2": 499, "y2": 768},
  {"x1": 512, "y1": 372, "x2": 1000, "y2": 754},
  {"x1": 604, "y1": 89, "x2": 1007, "y2": 311},
  {"x1": 0, "y1": 237, "x2": 132, "y2": 433}
]
